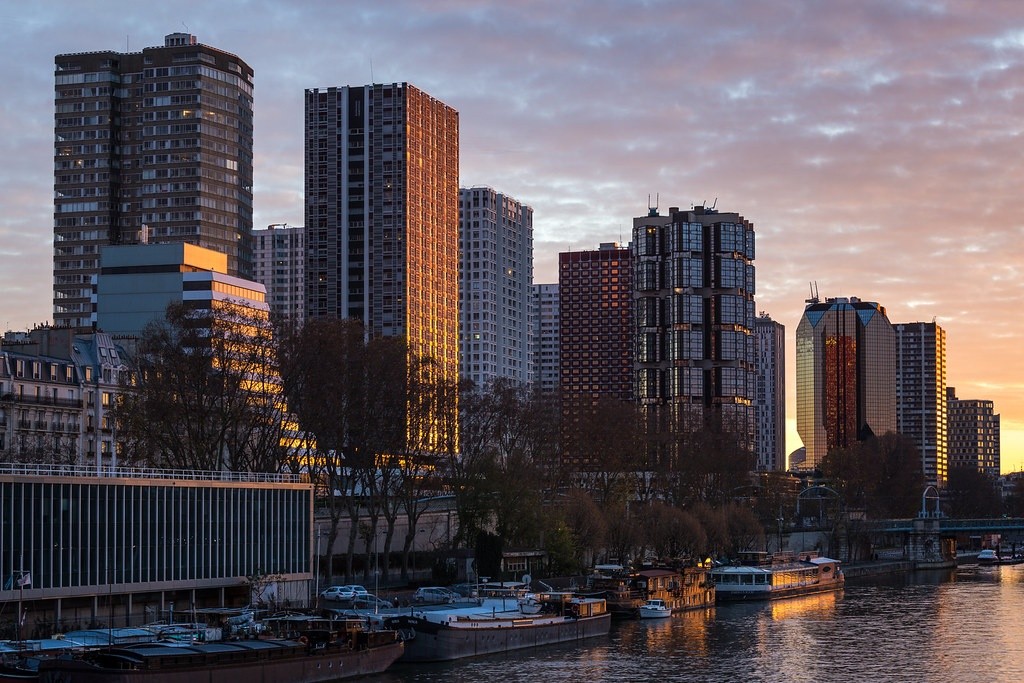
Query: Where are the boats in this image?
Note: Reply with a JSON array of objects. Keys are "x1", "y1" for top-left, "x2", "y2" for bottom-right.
[
  {"x1": 589, "y1": 565, "x2": 716, "y2": 612},
  {"x1": 383, "y1": 580, "x2": 612, "y2": 664},
  {"x1": 976, "y1": 549, "x2": 1024, "y2": 566},
  {"x1": 0, "y1": 583, "x2": 406, "y2": 682},
  {"x1": 709, "y1": 549, "x2": 845, "y2": 602},
  {"x1": 636, "y1": 598, "x2": 672, "y2": 619}
]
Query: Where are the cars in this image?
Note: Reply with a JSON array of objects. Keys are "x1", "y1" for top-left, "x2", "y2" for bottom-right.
[
  {"x1": 345, "y1": 585, "x2": 367, "y2": 596},
  {"x1": 319, "y1": 586, "x2": 354, "y2": 602},
  {"x1": 1, "y1": 606, "x2": 334, "y2": 641},
  {"x1": 413, "y1": 588, "x2": 451, "y2": 603},
  {"x1": 435, "y1": 587, "x2": 461, "y2": 599}
]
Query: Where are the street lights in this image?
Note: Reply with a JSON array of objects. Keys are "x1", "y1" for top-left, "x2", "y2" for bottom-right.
[{"x1": 776, "y1": 518, "x2": 785, "y2": 552}]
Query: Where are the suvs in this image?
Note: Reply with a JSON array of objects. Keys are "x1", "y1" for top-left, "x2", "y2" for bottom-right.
[{"x1": 349, "y1": 594, "x2": 393, "y2": 610}]
